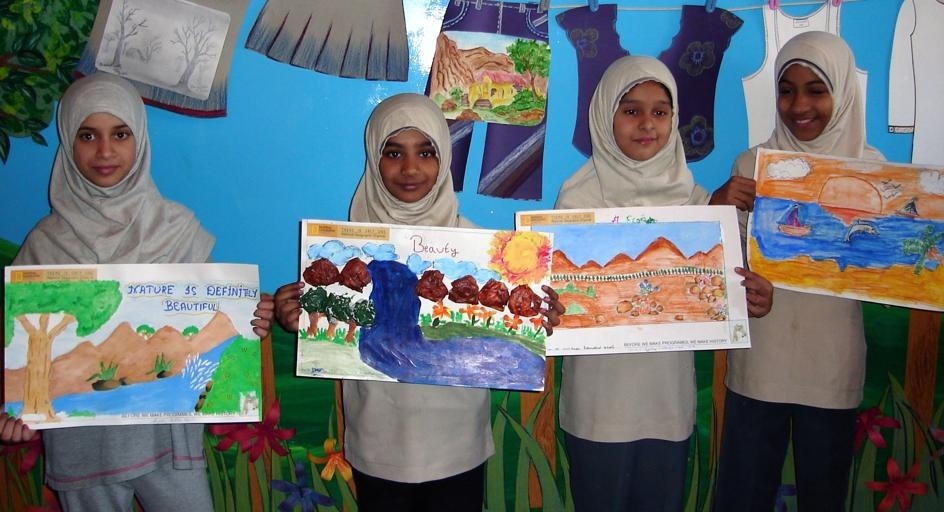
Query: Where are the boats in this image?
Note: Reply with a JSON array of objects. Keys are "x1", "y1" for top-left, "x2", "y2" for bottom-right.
[{"x1": 776, "y1": 203, "x2": 813, "y2": 240}]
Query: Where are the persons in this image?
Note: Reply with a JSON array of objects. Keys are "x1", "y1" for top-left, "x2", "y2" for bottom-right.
[
  {"x1": 554, "y1": 55, "x2": 773, "y2": 511},
  {"x1": 709, "y1": 31, "x2": 886, "y2": 512},
  {"x1": 275, "y1": 93, "x2": 565, "y2": 511},
  {"x1": 0, "y1": 73, "x2": 276, "y2": 512}
]
